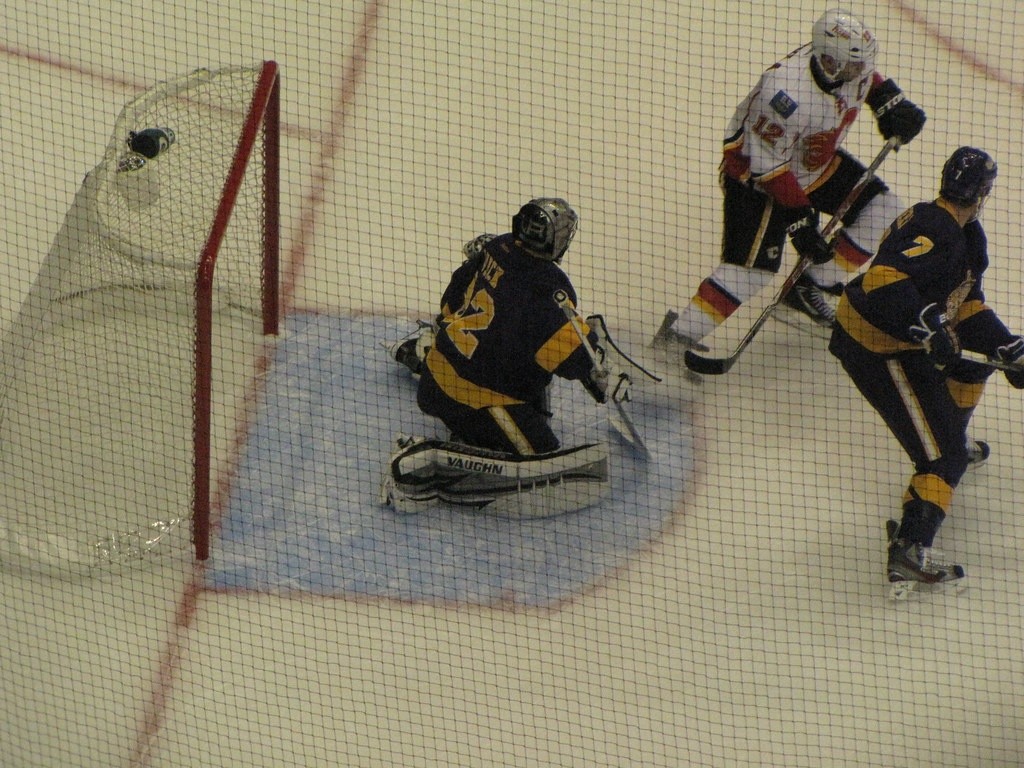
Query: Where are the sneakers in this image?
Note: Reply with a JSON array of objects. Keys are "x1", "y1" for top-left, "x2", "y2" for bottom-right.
[
  {"x1": 646, "y1": 311, "x2": 711, "y2": 384},
  {"x1": 964, "y1": 434, "x2": 990, "y2": 471},
  {"x1": 886, "y1": 521, "x2": 967, "y2": 601},
  {"x1": 771, "y1": 277, "x2": 837, "y2": 339}
]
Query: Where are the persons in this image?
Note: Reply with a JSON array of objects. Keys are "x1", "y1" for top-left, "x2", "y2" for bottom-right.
[
  {"x1": 647, "y1": 9, "x2": 928, "y2": 387},
  {"x1": 379, "y1": 197, "x2": 662, "y2": 517},
  {"x1": 829, "y1": 147, "x2": 1024, "y2": 601}
]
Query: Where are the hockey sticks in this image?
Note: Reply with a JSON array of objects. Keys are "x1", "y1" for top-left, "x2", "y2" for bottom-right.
[
  {"x1": 960, "y1": 347, "x2": 1024, "y2": 373},
  {"x1": 683, "y1": 134, "x2": 901, "y2": 376},
  {"x1": 561, "y1": 301, "x2": 653, "y2": 461}
]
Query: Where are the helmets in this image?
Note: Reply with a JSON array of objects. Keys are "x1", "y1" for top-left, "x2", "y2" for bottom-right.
[
  {"x1": 811, "y1": 9, "x2": 878, "y2": 63},
  {"x1": 511, "y1": 198, "x2": 578, "y2": 262},
  {"x1": 940, "y1": 145, "x2": 998, "y2": 203}
]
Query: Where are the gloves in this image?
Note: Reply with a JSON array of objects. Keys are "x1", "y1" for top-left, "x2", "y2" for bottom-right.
[
  {"x1": 867, "y1": 79, "x2": 926, "y2": 152},
  {"x1": 908, "y1": 302, "x2": 960, "y2": 385},
  {"x1": 787, "y1": 210, "x2": 836, "y2": 265},
  {"x1": 994, "y1": 336, "x2": 1024, "y2": 390}
]
